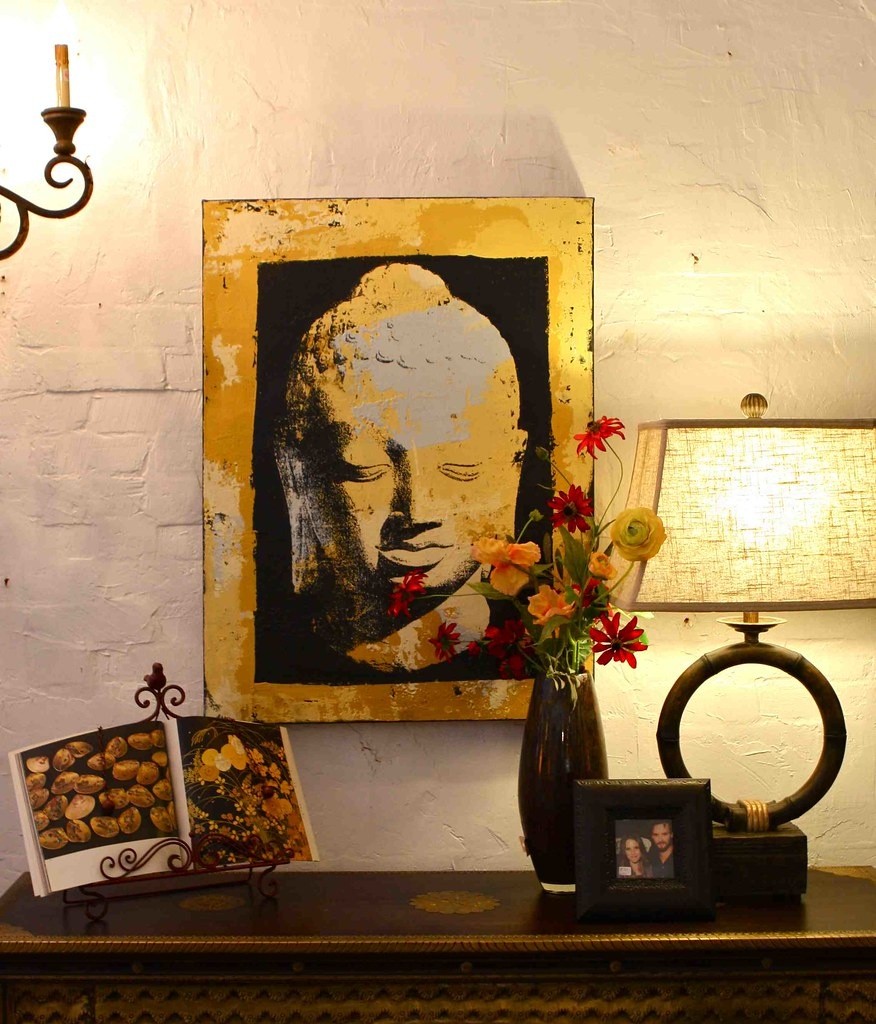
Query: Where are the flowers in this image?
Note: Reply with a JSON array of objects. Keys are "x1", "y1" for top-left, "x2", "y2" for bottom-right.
[{"x1": 388, "y1": 416, "x2": 666, "y2": 712}]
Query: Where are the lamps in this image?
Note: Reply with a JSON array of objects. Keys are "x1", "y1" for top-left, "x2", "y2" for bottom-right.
[
  {"x1": 598, "y1": 393, "x2": 876, "y2": 907},
  {"x1": 0, "y1": 41, "x2": 93, "y2": 261}
]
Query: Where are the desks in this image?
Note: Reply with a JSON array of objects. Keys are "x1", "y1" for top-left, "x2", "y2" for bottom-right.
[{"x1": 0, "y1": 864, "x2": 876, "y2": 1024}]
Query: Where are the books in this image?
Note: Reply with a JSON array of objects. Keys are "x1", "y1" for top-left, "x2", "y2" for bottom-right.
[{"x1": 9, "y1": 716, "x2": 320, "y2": 896}]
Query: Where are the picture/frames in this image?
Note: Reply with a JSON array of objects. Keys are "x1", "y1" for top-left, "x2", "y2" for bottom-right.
[
  {"x1": 573, "y1": 779, "x2": 716, "y2": 921},
  {"x1": 201, "y1": 198, "x2": 593, "y2": 722}
]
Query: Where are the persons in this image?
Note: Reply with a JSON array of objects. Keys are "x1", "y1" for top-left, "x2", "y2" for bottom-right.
[
  {"x1": 618, "y1": 833, "x2": 647, "y2": 878},
  {"x1": 283, "y1": 262, "x2": 528, "y2": 676},
  {"x1": 645, "y1": 821, "x2": 673, "y2": 879}
]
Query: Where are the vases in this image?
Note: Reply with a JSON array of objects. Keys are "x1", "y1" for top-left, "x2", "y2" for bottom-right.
[{"x1": 518, "y1": 670, "x2": 608, "y2": 895}]
